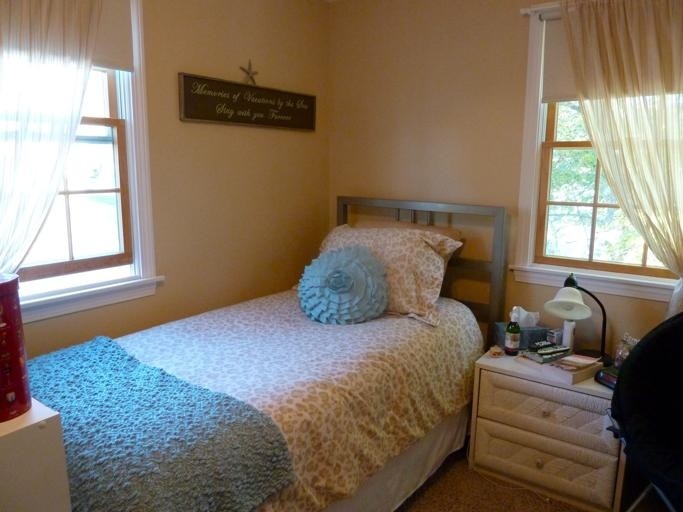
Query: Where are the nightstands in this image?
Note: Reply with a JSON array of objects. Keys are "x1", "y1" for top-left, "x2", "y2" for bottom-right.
[{"x1": 465, "y1": 345, "x2": 629, "y2": 512}]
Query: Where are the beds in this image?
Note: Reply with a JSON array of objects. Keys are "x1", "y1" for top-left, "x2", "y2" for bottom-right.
[{"x1": 25, "y1": 197, "x2": 511, "y2": 510}]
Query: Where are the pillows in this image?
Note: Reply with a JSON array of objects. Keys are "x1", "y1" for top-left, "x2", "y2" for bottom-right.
[{"x1": 287, "y1": 214, "x2": 466, "y2": 325}]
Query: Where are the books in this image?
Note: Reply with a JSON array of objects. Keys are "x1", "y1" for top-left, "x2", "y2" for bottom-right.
[
  {"x1": 514, "y1": 340, "x2": 621, "y2": 391},
  {"x1": 518, "y1": 341, "x2": 574, "y2": 366}
]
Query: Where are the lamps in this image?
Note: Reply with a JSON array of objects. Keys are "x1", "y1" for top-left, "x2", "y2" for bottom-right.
[{"x1": 544, "y1": 274, "x2": 613, "y2": 365}]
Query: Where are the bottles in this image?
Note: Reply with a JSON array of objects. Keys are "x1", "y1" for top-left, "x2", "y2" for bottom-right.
[
  {"x1": 504, "y1": 312, "x2": 521, "y2": 356},
  {"x1": 560, "y1": 318, "x2": 575, "y2": 352}
]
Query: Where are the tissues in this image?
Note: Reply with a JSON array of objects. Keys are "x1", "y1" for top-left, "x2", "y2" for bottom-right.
[{"x1": 495, "y1": 304, "x2": 548, "y2": 350}]
